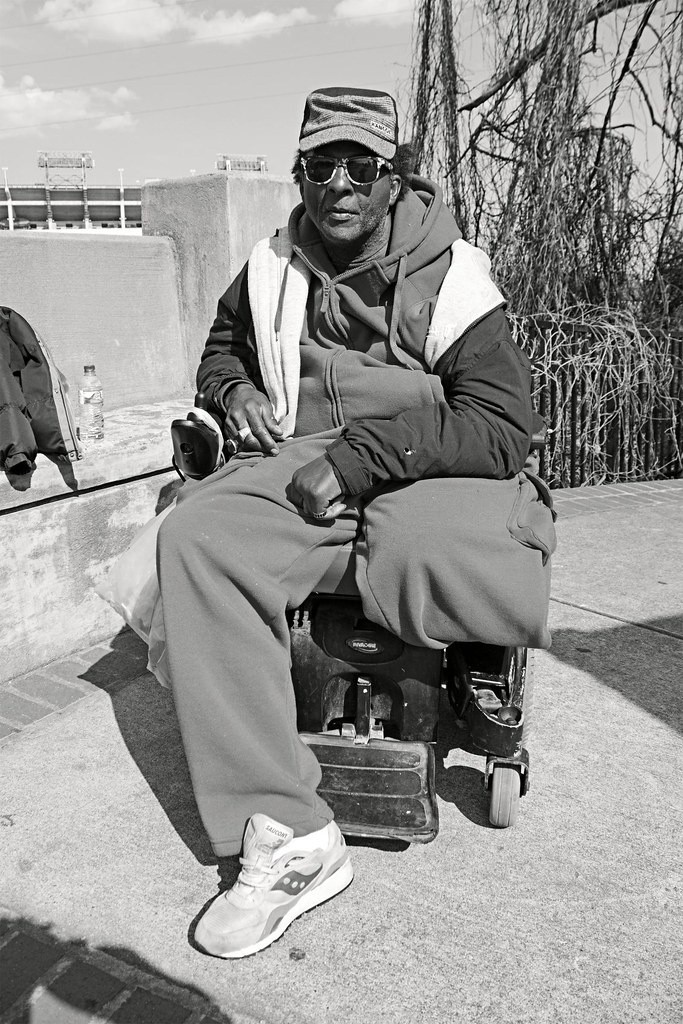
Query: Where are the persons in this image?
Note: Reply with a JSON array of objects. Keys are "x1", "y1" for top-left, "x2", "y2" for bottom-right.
[{"x1": 155, "y1": 87, "x2": 558, "y2": 959}]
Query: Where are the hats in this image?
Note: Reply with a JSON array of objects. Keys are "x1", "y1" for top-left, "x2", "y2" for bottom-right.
[{"x1": 299, "y1": 87, "x2": 398, "y2": 160}]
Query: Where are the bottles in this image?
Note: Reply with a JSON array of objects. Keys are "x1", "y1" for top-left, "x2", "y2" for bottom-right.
[{"x1": 78, "y1": 365, "x2": 105, "y2": 443}]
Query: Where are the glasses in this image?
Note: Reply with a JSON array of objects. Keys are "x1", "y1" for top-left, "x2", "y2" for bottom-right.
[{"x1": 300, "y1": 154, "x2": 394, "y2": 186}]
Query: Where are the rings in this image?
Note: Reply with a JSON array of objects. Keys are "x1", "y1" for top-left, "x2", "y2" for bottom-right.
[
  {"x1": 225, "y1": 439, "x2": 240, "y2": 454},
  {"x1": 238, "y1": 428, "x2": 251, "y2": 443},
  {"x1": 311, "y1": 510, "x2": 329, "y2": 519}
]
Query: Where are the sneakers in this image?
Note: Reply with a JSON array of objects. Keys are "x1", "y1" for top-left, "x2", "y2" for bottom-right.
[{"x1": 193, "y1": 813, "x2": 354, "y2": 959}]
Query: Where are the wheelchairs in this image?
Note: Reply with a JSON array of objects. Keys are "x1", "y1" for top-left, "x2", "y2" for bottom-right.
[{"x1": 156, "y1": 391, "x2": 547, "y2": 847}]
